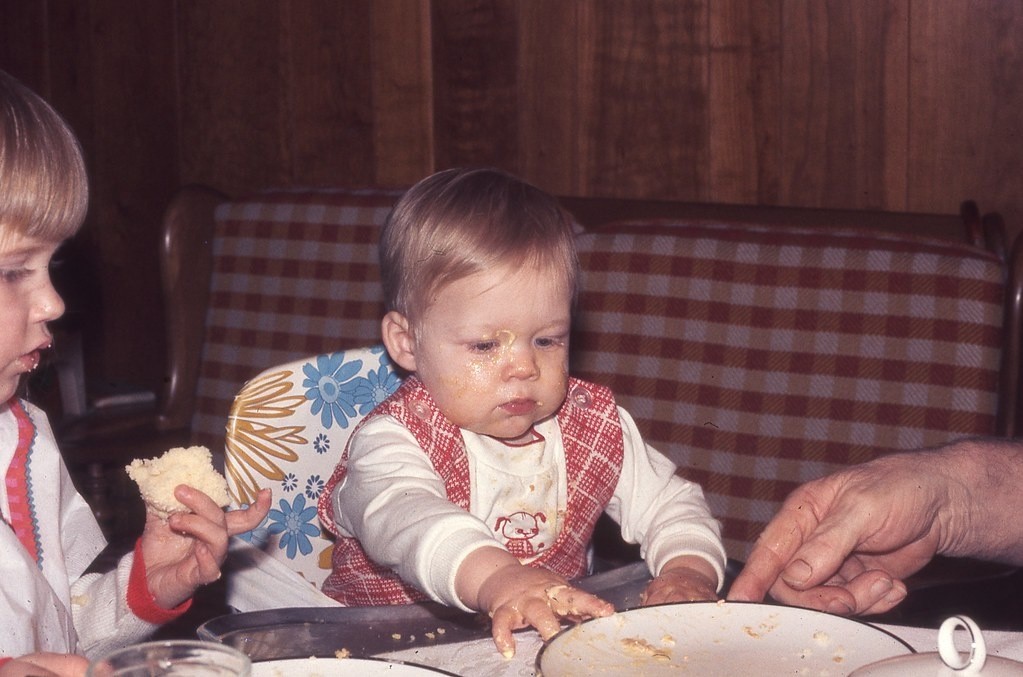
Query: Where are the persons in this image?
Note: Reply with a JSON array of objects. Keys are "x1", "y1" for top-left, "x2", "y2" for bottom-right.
[
  {"x1": 2, "y1": 72, "x2": 272, "y2": 675},
  {"x1": 317, "y1": 169, "x2": 728, "y2": 656},
  {"x1": 728, "y1": 433, "x2": 1022, "y2": 616}
]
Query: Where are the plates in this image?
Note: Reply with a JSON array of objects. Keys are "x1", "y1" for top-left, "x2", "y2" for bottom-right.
[
  {"x1": 534, "y1": 599, "x2": 923, "y2": 677},
  {"x1": 248, "y1": 657, "x2": 461, "y2": 677}
]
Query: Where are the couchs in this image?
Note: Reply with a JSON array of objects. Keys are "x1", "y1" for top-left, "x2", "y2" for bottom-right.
[{"x1": 47, "y1": 180, "x2": 1023, "y2": 624}]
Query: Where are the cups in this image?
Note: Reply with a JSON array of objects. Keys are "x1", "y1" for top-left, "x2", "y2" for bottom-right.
[{"x1": 84, "y1": 638, "x2": 253, "y2": 677}]
